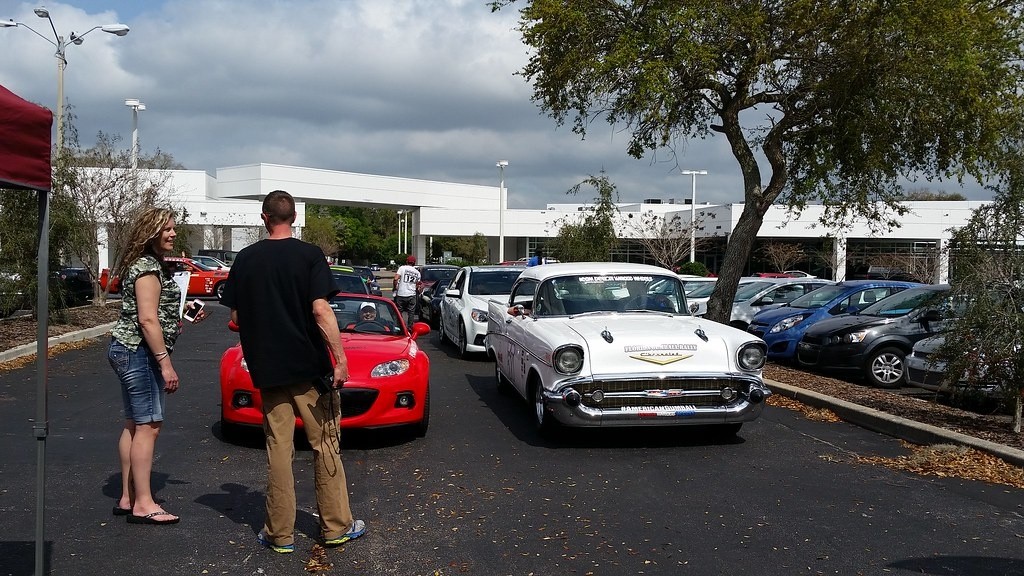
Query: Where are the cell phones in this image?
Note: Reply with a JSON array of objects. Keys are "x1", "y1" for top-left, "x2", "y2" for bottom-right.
[{"x1": 183, "y1": 298, "x2": 205, "y2": 322}]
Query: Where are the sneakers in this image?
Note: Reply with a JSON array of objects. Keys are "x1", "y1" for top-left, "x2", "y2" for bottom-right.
[
  {"x1": 256, "y1": 528, "x2": 294, "y2": 553},
  {"x1": 325, "y1": 520, "x2": 365, "y2": 544}
]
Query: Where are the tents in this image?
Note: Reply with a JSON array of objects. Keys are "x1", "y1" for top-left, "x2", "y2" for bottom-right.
[{"x1": 0, "y1": 85, "x2": 53, "y2": 576}]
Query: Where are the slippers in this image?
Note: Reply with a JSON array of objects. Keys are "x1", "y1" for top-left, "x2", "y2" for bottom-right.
[
  {"x1": 113, "y1": 506, "x2": 133, "y2": 516},
  {"x1": 127, "y1": 512, "x2": 179, "y2": 524}
]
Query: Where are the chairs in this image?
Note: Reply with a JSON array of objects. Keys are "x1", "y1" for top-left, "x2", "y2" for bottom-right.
[{"x1": 858, "y1": 291, "x2": 876, "y2": 310}]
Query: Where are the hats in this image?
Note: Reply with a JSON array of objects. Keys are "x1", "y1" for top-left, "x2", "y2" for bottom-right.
[
  {"x1": 406, "y1": 256, "x2": 416, "y2": 263},
  {"x1": 360, "y1": 302, "x2": 376, "y2": 312}
]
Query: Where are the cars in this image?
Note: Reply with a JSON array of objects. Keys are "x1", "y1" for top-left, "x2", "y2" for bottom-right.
[
  {"x1": 328, "y1": 264, "x2": 384, "y2": 296},
  {"x1": 797, "y1": 284, "x2": 1000, "y2": 390},
  {"x1": 427, "y1": 265, "x2": 565, "y2": 358},
  {"x1": 746, "y1": 279, "x2": 930, "y2": 366},
  {"x1": 417, "y1": 277, "x2": 453, "y2": 331},
  {"x1": 602, "y1": 264, "x2": 923, "y2": 331},
  {"x1": 99, "y1": 255, "x2": 235, "y2": 301},
  {"x1": 903, "y1": 309, "x2": 1024, "y2": 416},
  {"x1": 0, "y1": 258, "x2": 94, "y2": 318},
  {"x1": 413, "y1": 265, "x2": 462, "y2": 306}
]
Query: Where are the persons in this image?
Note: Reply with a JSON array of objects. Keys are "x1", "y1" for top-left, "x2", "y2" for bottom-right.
[
  {"x1": 507, "y1": 301, "x2": 533, "y2": 316},
  {"x1": 108, "y1": 209, "x2": 205, "y2": 526},
  {"x1": 218, "y1": 191, "x2": 366, "y2": 552},
  {"x1": 347, "y1": 302, "x2": 390, "y2": 332},
  {"x1": 393, "y1": 256, "x2": 421, "y2": 333}
]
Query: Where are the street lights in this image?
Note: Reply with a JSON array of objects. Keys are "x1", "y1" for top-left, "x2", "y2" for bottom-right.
[
  {"x1": 0, "y1": 7, "x2": 130, "y2": 196},
  {"x1": 495, "y1": 159, "x2": 509, "y2": 263},
  {"x1": 681, "y1": 169, "x2": 708, "y2": 262},
  {"x1": 124, "y1": 98, "x2": 146, "y2": 211}
]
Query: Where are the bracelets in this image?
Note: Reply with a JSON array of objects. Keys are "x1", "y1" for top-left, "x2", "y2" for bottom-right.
[{"x1": 155, "y1": 351, "x2": 168, "y2": 362}]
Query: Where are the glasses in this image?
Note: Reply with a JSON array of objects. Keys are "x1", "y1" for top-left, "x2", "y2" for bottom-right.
[{"x1": 363, "y1": 309, "x2": 374, "y2": 313}]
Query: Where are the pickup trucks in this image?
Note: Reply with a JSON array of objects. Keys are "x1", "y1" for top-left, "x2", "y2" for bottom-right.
[{"x1": 483, "y1": 262, "x2": 771, "y2": 438}]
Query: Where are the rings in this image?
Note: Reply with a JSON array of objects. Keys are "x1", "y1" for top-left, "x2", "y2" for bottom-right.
[{"x1": 173, "y1": 385, "x2": 176, "y2": 386}]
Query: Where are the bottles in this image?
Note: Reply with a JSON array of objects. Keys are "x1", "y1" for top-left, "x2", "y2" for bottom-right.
[{"x1": 393, "y1": 290, "x2": 397, "y2": 297}]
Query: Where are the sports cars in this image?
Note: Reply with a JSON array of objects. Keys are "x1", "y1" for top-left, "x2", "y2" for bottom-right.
[{"x1": 220, "y1": 293, "x2": 432, "y2": 437}]
